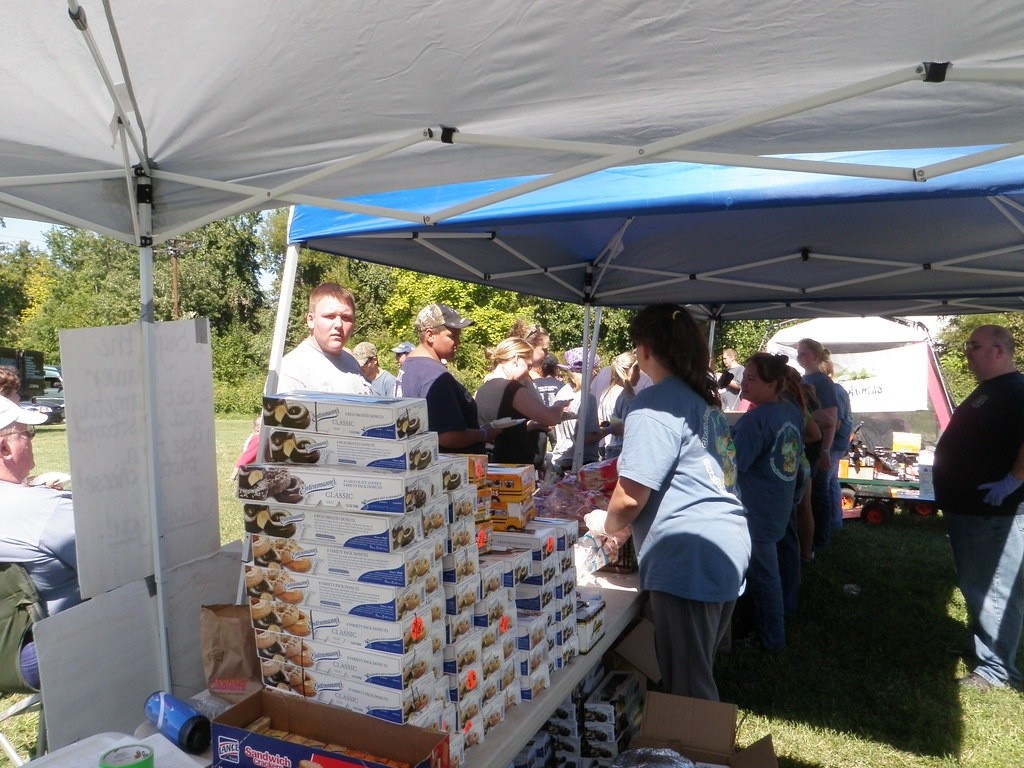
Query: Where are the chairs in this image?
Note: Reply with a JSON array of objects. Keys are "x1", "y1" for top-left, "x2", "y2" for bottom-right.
[{"x1": 0, "y1": 562, "x2": 49, "y2": 768}]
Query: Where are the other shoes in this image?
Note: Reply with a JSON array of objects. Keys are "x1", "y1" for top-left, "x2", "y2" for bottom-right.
[
  {"x1": 952, "y1": 671, "x2": 990, "y2": 687},
  {"x1": 948, "y1": 646, "x2": 974, "y2": 658}
]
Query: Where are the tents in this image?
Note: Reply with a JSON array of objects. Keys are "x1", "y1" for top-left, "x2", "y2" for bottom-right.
[
  {"x1": 0, "y1": 0, "x2": 1024, "y2": 706},
  {"x1": 235, "y1": 144, "x2": 1024, "y2": 598}
]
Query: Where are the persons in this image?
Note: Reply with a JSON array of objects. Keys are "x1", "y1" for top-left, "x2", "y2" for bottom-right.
[
  {"x1": 356, "y1": 322, "x2": 652, "y2": 485},
  {"x1": 231, "y1": 416, "x2": 261, "y2": 481},
  {"x1": 401, "y1": 305, "x2": 502, "y2": 454},
  {"x1": 933, "y1": 325, "x2": 1024, "y2": 687},
  {"x1": 263, "y1": 284, "x2": 371, "y2": 396},
  {"x1": 0, "y1": 365, "x2": 82, "y2": 690},
  {"x1": 712, "y1": 338, "x2": 851, "y2": 653},
  {"x1": 583, "y1": 303, "x2": 750, "y2": 703}
]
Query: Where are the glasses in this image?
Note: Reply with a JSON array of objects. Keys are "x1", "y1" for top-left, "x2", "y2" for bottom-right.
[
  {"x1": 0, "y1": 425, "x2": 35, "y2": 440},
  {"x1": 963, "y1": 341, "x2": 1001, "y2": 352},
  {"x1": 525, "y1": 325, "x2": 540, "y2": 339}
]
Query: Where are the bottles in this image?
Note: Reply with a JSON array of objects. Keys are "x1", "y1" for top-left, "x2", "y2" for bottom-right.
[{"x1": 144, "y1": 691, "x2": 212, "y2": 755}]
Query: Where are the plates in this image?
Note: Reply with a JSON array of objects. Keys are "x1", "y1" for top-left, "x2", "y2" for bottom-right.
[{"x1": 481, "y1": 417, "x2": 527, "y2": 427}]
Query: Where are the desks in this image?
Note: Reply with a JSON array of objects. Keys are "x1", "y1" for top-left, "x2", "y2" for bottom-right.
[
  {"x1": 132, "y1": 570, "x2": 649, "y2": 768},
  {"x1": 17, "y1": 732, "x2": 137, "y2": 768}
]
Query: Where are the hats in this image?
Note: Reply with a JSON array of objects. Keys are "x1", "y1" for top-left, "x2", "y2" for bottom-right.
[
  {"x1": 0, "y1": 394, "x2": 48, "y2": 430},
  {"x1": 390, "y1": 341, "x2": 416, "y2": 353},
  {"x1": 352, "y1": 341, "x2": 378, "y2": 368},
  {"x1": 415, "y1": 304, "x2": 475, "y2": 329}
]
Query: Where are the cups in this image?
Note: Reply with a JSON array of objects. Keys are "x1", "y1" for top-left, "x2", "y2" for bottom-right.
[{"x1": 839, "y1": 458, "x2": 849, "y2": 479}]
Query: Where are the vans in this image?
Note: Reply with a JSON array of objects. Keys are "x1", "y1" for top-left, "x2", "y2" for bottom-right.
[{"x1": 19, "y1": 365, "x2": 66, "y2": 421}]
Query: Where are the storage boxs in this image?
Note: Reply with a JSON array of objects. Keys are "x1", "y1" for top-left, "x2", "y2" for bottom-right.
[
  {"x1": 837, "y1": 432, "x2": 936, "y2": 499},
  {"x1": 211, "y1": 388, "x2": 780, "y2": 768}
]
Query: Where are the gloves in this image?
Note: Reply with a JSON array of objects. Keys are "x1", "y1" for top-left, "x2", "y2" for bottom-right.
[{"x1": 977, "y1": 472, "x2": 1024, "y2": 506}]
